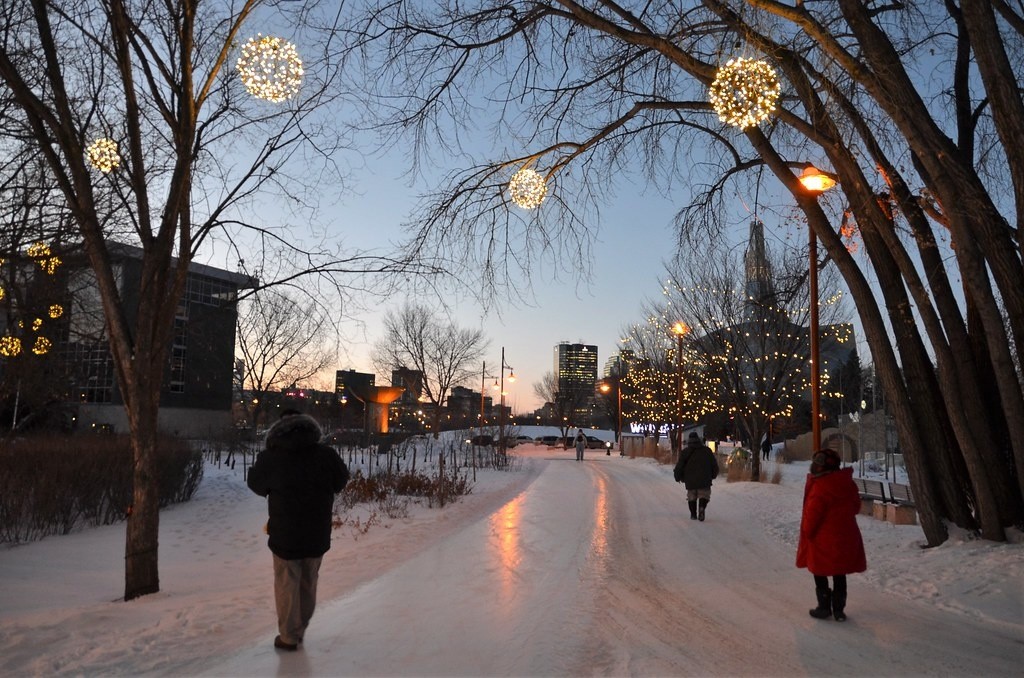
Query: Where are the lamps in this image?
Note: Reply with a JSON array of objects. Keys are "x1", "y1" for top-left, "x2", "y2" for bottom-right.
[{"x1": 796, "y1": 160, "x2": 838, "y2": 192}]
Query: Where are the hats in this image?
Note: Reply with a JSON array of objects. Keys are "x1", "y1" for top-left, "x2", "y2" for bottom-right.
[{"x1": 810, "y1": 447, "x2": 840, "y2": 474}]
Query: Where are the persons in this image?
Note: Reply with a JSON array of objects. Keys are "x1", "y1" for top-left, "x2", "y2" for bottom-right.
[
  {"x1": 795, "y1": 449, "x2": 867, "y2": 622},
  {"x1": 248, "y1": 408, "x2": 350, "y2": 651},
  {"x1": 761, "y1": 437, "x2": 773, "y2": 461},
  {"x1": 573, "y1": 429, "x2": 588, "y2": 461},
  {"x1": 673, "y1": 431, "x2": 720, "y2": 521},
  {"x1": 121, "y1": 504, "x2": 134, "y2": 522}
]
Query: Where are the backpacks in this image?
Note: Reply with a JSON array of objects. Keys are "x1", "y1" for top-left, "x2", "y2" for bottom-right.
[{"x1": 578, "y1": 434, "x2": 583, "y2": 442}]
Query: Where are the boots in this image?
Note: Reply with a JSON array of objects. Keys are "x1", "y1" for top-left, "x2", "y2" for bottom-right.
[
  {"x1": 832, "y1": 591, "x2": 847, "y2": 621},
  {"x1": 699, "y1": 498, "x2": 709, "y2": 521},
  {"x1": 810, "y1": 587, "x2": 832, "y2": 619},
  {"x1": 689, "y1": 500, "x2": 697, "y2": 520}
]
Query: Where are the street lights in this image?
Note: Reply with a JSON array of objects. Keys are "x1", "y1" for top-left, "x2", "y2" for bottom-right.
[
  {"x1": 802, "y1": 164, "x2": 838, "y2": 454},
  {"x1": 601, "y1": 376, "x2": 623, "y2": 455},
  {"x1": 480, "y1": 360, "x2": 499, "y2": 443},
  {"x1": 673, "y1": 322, "x2": 688, "y2": 461},
  {"x1": 498, "y1": 346, "x2": 514, "y2": 455}
]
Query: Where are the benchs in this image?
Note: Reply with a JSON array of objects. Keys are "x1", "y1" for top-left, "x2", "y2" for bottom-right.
[{"x1": 851, "y1": 477, "x2": 921, "y2": 525}]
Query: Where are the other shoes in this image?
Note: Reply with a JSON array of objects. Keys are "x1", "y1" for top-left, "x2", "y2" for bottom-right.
[{"x1": 274, "y1": 635, "x2": 297, "y2": 651}]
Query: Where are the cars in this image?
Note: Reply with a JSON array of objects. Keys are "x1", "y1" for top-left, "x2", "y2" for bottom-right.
[
  {"x1": 501, "y1": 434, "x2": 607, "y2": 450},
  {"x1": 465, "y1": 436, "x2": 495, "y2": 448}
]
